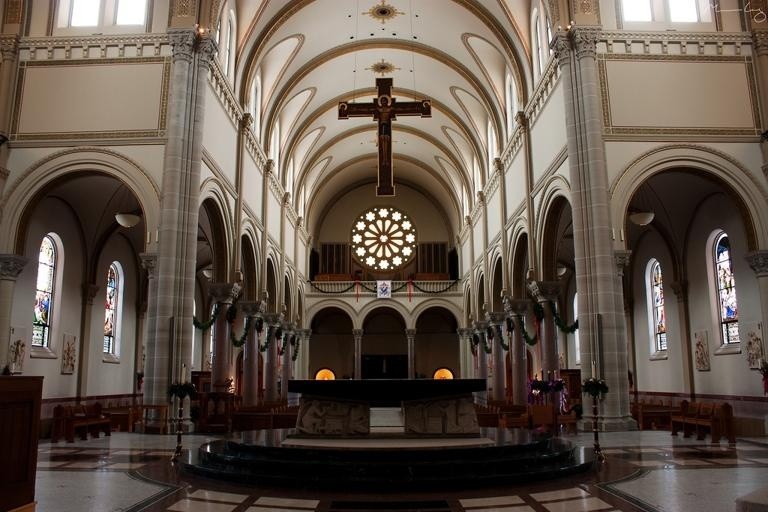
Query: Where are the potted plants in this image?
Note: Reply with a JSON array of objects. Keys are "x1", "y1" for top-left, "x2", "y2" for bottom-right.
[
  {"x1": 167, "y1": 381, "x2": 197, "y2": 399},
  {"x1": 580, "y1": 376, "x2": 609, "y2": 463}
]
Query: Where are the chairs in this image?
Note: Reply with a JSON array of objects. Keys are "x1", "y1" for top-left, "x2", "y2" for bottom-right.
[
  {"x1": 473, "y1": 400, "x2": 559, "y2": 436},
  {"x1": 51, "y1": 401, "x2": 142, "y2": 443},
  {"x1": 630, "y1": 398, "x2": 736, "y2": 443}
]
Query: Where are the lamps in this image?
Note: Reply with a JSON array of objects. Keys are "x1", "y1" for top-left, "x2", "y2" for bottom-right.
[
  {"x1": 556, "y1": 266, "x2": 567, "y2": 277},
  {"x1": 202, "y1": 269, "x2": 212, "y2": 278},
  {"x1": 113, "y1": 209, "x2": 143, "y2": 228},
  {"x1": 629, "y1": 209, "x2": 656, "y2": 226}
]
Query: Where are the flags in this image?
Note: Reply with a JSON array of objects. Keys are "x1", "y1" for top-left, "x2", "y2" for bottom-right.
[{"x1": 374, "y1": 279, "x2": 393, "y2": 299}]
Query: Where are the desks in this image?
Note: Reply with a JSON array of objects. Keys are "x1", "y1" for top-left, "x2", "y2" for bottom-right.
[
  {"x1": 288, "y1": 379, "x2": 487, "y2": 438},
  {"x1": 196, "y1": 392, "x2": 234, "y2": 432},
  {"x1": 142, "y1": 405, "x2": 168, "y2": 434}
]
